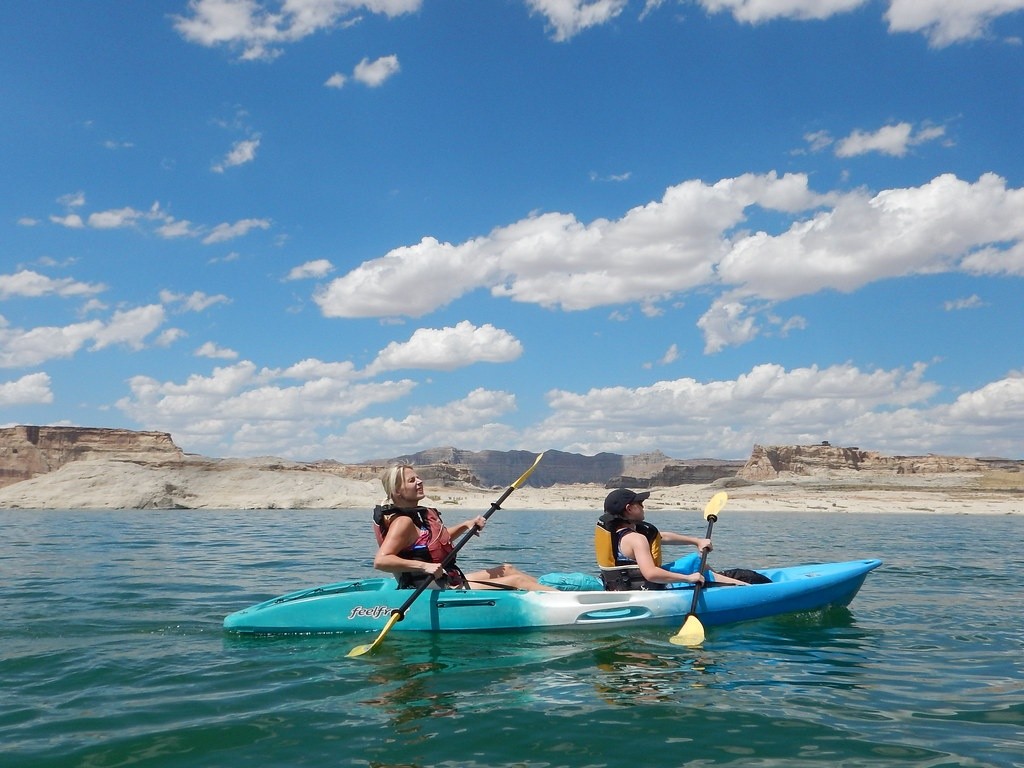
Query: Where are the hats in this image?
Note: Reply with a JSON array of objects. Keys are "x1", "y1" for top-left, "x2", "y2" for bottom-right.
[{"x1": 604, "y1": 489, "x2": 650, "y2": 515}]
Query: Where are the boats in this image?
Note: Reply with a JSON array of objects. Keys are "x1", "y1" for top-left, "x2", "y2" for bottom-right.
[{"x1": 222, "y1": 558, "x2": 883, "y2": 634}]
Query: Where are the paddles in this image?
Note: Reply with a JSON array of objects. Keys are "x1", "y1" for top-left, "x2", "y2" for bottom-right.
[
  {"x1": 343, "y1": 452, "x2": 543, "y2": 659},
  {"x1": 670, "y1": 491, "x2": 728, "y2": 638}
]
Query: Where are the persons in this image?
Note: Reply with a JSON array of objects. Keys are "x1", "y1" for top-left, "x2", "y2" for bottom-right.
[
  {"x1": 595, "y1": 490, "x2": 750, "y2": 591},
  {"x1": 373, "y1": 464, "x2": 558, "y2": 590}
]
